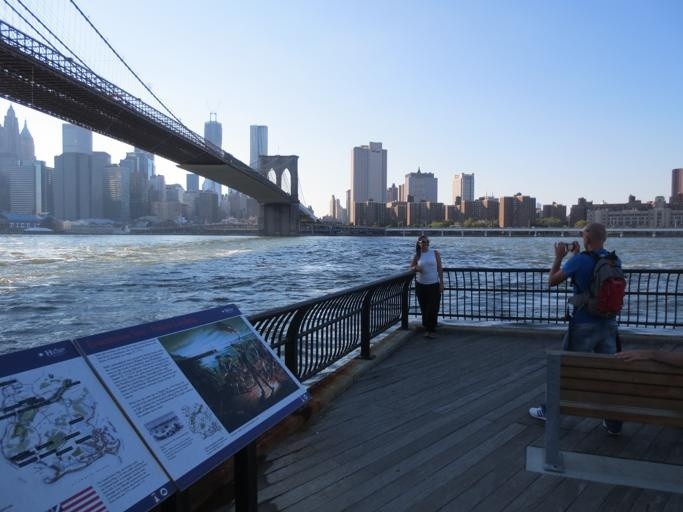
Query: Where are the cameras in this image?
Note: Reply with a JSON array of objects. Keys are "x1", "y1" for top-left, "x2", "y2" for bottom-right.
[{"x1": 558, "y1": 243, "x2": 575, "y2": 254}]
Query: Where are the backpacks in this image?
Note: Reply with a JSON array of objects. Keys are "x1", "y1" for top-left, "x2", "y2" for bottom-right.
[{"x1": 570, "y1": 250, "x2": 626, "y2": 320}]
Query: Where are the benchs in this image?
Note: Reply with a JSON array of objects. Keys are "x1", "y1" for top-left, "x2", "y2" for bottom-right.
[{"x1": 541, "y1": 348, "x2": 683, "y2": 472}]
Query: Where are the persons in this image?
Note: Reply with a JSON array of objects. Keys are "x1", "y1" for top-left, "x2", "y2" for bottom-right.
[
  {"x1": 194, "y1": 329, "x2": 298, "y2": 432},
  {"x1": 410, "y1": 234, "x2": 445, "y2": 340},
  {"x1": 613, "y1": 349, "x2": 682, "y2": 371},
  {"x1": 526, "y1": 222, "x2": 626, "y2": 436}
]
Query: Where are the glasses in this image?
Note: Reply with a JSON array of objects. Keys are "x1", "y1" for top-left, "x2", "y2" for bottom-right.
[{"x1": 419, "y1": 240, "x2": 429, "y2": 243}]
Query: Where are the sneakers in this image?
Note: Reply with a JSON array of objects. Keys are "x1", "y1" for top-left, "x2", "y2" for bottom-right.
[
  {"x1": 423, "y1": 329, "x2": 436, "y2": 339},
  {"x1": 602, "y1": 418, "x2": 621, "y2": 436},
  {"x1": 529, "y1": 407, "x2": 546, "y2": 421}
]
